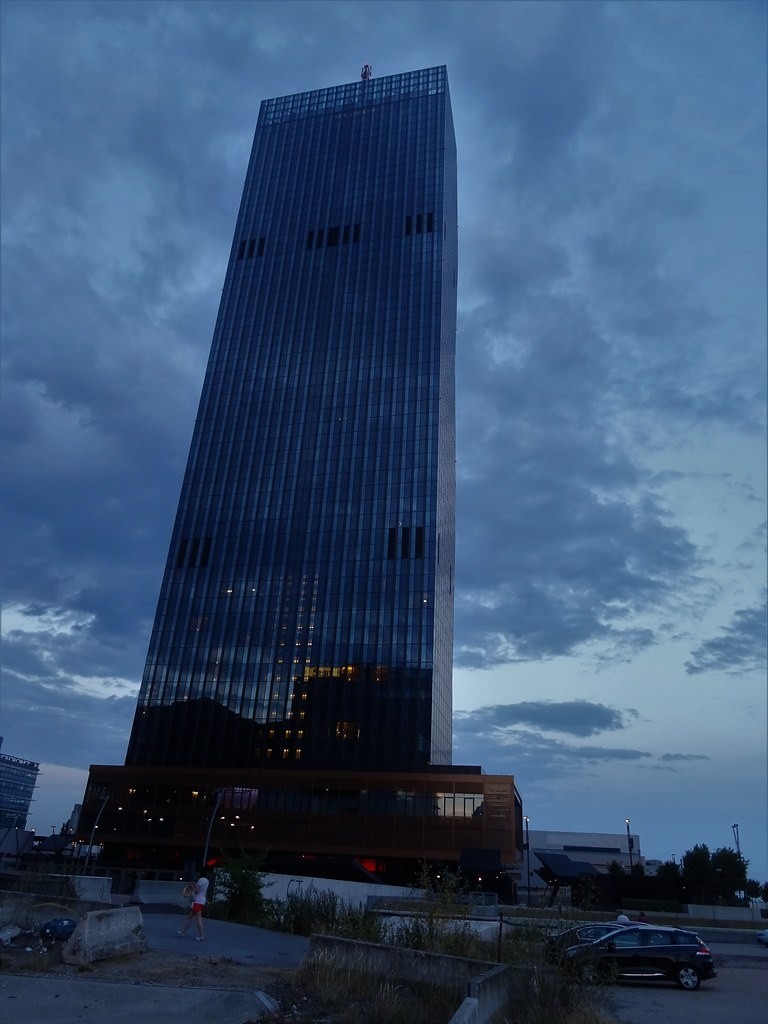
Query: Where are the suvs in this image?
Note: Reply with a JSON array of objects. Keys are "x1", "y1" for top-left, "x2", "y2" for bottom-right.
[{"x1": 558, "y1": 926, "x2": 718, "y2": 991}]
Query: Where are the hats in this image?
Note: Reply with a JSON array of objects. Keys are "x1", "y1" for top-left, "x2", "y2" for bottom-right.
[{"x1": 183, "y1": 885, "x2": 192, "y2": 897}]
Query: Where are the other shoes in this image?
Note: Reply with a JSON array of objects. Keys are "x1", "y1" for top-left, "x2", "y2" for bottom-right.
[
  {"x1": 196, "y1": 937, "x2": 201, "y2": 941},
  {"x1": 177, "y1": 930, "x2": 184, "y2": 936}
]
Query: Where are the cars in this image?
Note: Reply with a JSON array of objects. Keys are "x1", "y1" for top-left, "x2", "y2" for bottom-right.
[
  {"x1": 756, "y1": 929, "x2": 768, "y2": 948},
  {"x1": 584, "y1": 921, "x2": 662, "y2": 939},
  {"x1": 547, "y1": 923, "x2": 653, "y2": 966}
]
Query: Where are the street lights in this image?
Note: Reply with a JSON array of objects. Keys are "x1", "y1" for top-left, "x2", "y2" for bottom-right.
[
  {"x1": 625, "y1": 818, "x2": 633, "y2": 870},
  {"x1": 732, "y1": 824, "x2": 741, "y2": 861},
  {"x1": 523, "y1": 815, "x2": 531, "y2": 908},
  {"x1": 81, "y1": 792, "x2": 115, "y2": 877},
  {"x1": 201, "y1": 787, "x2": 228, "y2": 876}
]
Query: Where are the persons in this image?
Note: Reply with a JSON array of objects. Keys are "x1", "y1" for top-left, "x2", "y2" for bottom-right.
[
  {"x1": 176, "y1": 866, "x2": 211, "y2": 941},
  {"x1": 617, "y1": 911, "x2": 631, "y2": 922},
  {"x1": 638, "y1": 911, "x2": 648, "y2": 923}
]
